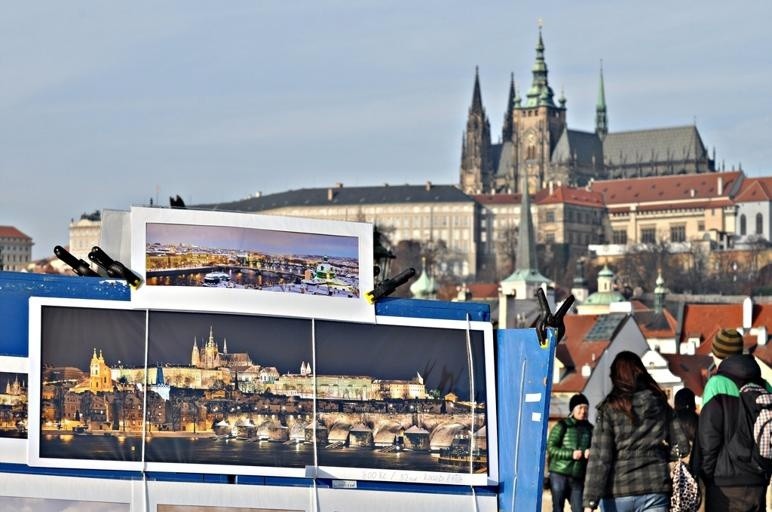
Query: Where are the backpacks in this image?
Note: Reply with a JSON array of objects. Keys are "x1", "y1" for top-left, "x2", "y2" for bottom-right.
[{"x1": 715, "y1": 372, "x2": 772, "y2": 472}]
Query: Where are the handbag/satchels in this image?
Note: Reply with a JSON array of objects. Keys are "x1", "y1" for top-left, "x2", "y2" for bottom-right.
[{"x1": 670, "y1": 459, "x2": 704, "y2": 512}]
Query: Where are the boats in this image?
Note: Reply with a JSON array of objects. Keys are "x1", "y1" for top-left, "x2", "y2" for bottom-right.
[
  {"x1": 439, "y1": 434, "x2": 488, "y2": 469},
  {"x1": 200, "y1": 271, "x2": 232, "y2": 287}
]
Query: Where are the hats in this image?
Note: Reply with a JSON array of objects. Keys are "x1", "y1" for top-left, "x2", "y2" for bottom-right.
[
  {"x1": 710, "y1": 327, "x2": 744, "y2": 359},
  {"x1": 569, "y1": 393, "x2": 589, "y2": 411}
]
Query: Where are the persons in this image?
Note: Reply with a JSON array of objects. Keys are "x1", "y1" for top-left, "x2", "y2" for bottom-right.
[
  {"x1": 581, "y1": 350, "x2": 689, "y2": 512},
  {"x1": 673, "y1": 387, "x2": 700, "y2": 440},
  {"x1": 688, "y1": 328, "x2": 771, "y2": 512},
  {"x1": 547, "y1": 393, "x2": 595, "y2": 512}
]
